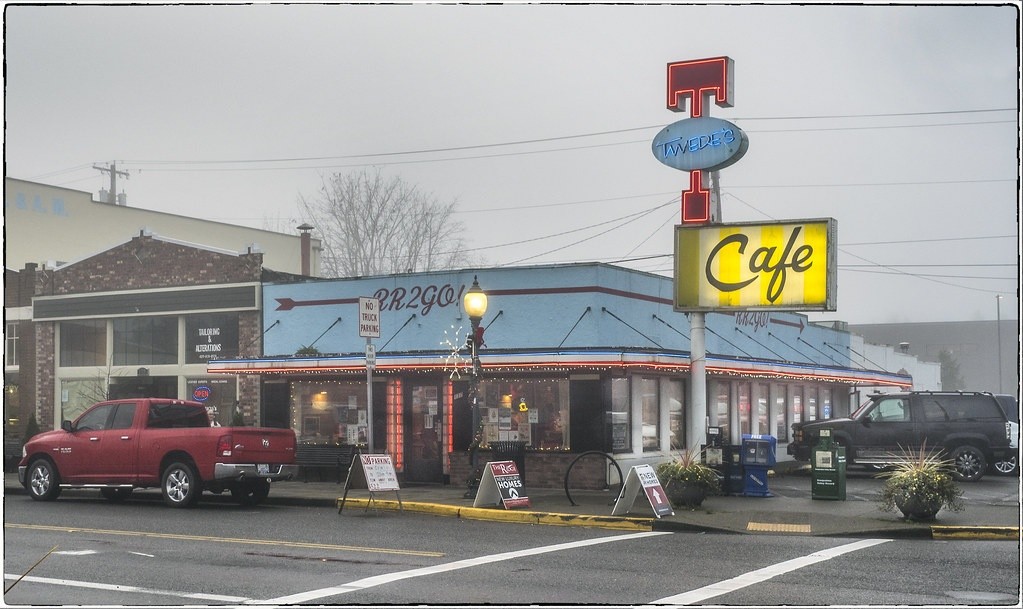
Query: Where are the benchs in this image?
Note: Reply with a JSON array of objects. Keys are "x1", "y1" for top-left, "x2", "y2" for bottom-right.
[{"x1": 292, "y1": 443, "x2": 357, "y2": 484}]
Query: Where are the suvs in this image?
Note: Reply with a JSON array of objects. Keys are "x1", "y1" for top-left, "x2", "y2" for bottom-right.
[
  {"x1": 786, "y1": 389, "x2": 1011, "y2": 484},
  {"x1": 979, "y1": 394, "x2": 1019, "y2": 475}
]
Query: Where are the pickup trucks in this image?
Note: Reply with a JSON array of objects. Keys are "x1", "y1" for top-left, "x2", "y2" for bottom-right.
[{"x1": 15, "y1": 398, "x2": 297, "y2": 511}]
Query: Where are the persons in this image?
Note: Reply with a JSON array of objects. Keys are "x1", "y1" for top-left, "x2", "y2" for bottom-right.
[{"x1": 207, "y1": 410, "x2": 222, "y2": 427}]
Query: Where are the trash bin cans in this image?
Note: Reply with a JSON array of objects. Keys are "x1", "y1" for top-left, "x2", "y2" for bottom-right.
[
  {"x1": 699, "y1": 443, "x2": 743, "y2": 498},
  {"x1": 739, "y1": 434, "x2": 778, "y2": 498},
  {"x1": 810, "y1": 444, "x2": 847, "y2": 501}
]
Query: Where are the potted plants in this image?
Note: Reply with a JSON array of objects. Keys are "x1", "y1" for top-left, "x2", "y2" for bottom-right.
[
  {"x1": 653, "y1": 432, "x2": 722, "y2": 511},
  {"x1": 871, "y1": 435, "x2": 966, "y2": 522}
]
